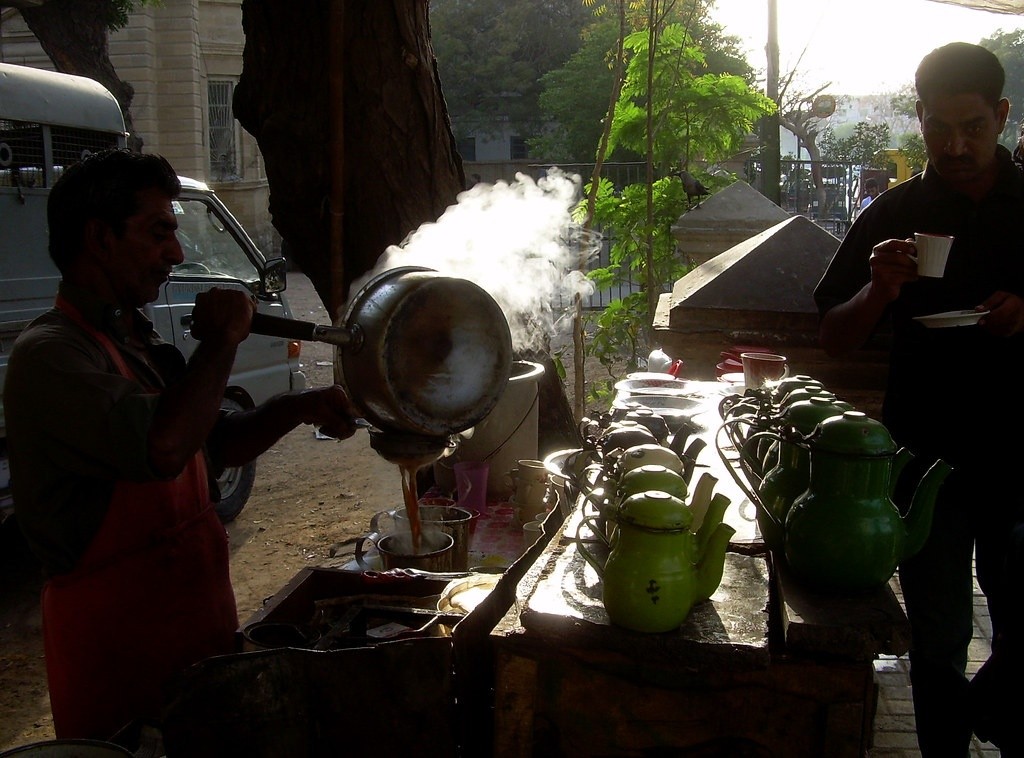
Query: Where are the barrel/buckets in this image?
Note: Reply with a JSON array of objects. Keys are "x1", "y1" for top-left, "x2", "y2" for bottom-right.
[
  {"x1": 433, "y1": 360, "x2": 545, "y2": 494},
  {"x1": 242, "y1": 620, "x2": 320, "y2": 652}
]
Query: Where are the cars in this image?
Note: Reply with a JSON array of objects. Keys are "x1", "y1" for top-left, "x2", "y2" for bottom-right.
[{"x1": 780, "y1": 164, "x2": 862, "y2": 193}]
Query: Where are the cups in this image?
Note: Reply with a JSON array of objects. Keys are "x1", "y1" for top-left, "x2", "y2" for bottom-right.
[
  {"x1": 371, "y1": 507, "x2": 472, "y2": 572},
  {"x1": 355, "y1": 532, "x2": 453, "y2": 574},
  {"x1": 417, "y1": 498, "x2": 456, "y2": 507},
  {"x1": 741, "y1": 353, "x2": 790, "y2": 390},
  {"x1": 905, "y1": 233, "x2": 955, "y2": 277},
  {"x1": 454, "y1": 462, "x2": 488, "y2": 515},
  {"x1": 456, "y1": 507, "x2": 480, "y2": 535},
  {"x1": 503, "y1": 460, "x2": 565, "y2": 550}
]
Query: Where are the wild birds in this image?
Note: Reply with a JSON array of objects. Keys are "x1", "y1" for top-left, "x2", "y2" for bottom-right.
[{"x1": 673, "y1": 170, "x2": 713, "y2": 211}]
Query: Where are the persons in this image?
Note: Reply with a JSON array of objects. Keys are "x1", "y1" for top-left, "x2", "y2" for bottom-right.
[
  {"x1": 6, "y1": 148, "x2": 356, "y2": 741},
  {"x1": 813, "y1": 45, "x2": 1024, "y2": 758}
]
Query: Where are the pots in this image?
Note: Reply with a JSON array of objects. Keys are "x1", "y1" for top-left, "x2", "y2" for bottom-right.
[{"x1": 179, "y1": 265, "x2": 513, "y2": 434}]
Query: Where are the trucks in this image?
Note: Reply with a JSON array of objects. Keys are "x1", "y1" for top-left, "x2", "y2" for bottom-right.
[{"x1": 1, "y1": 64, "x2": 298, "y2": 527}]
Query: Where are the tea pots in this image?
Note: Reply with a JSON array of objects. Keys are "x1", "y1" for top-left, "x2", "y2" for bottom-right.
[{"x1": 565, "y1": 375, "x2": 950, "y2": 630}]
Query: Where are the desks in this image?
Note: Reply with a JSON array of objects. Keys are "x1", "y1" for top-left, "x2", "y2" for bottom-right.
[{"x1": 514, "y1": 369, "x2": 917, "y2": 758}]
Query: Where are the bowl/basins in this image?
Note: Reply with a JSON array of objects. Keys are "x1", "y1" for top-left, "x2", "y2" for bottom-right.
[{"x1": 0, "y1": 740, "x2": 134, "y2": 758}]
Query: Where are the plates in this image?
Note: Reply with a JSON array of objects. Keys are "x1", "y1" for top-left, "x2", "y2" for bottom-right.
[
  {"x1": 912, "y1": 310, "x2": 990, "y2": 328},
  {"x1": 613, "y1": 396, "x2": 710, "y2": 420},
  {"x1": 626, "y1": 372, "x2": 675, "y2": 381},
  {"x1": 614, "y1": 380, "x2": 699, "y2": 396}
]
